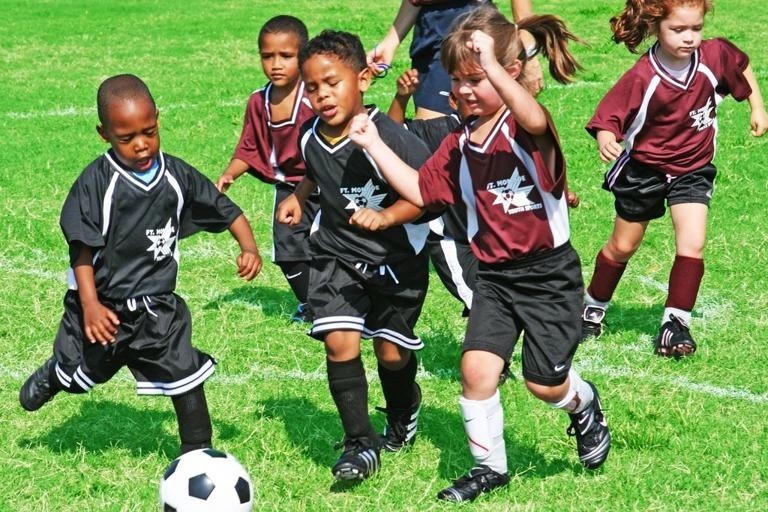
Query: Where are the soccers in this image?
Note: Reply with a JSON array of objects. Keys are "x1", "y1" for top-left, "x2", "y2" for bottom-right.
[{"x1": 157, "y1": 448, "x2": 253, "y2": 512}]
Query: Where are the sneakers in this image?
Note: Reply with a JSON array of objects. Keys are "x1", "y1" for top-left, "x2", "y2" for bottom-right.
[
  {"x1": 332, "y1": 380, "x2": 422, "y2": 480},
  {"x1": 657, "y1": 314, "x2": 696, "y2": 356},
  {"x1": 19, "y1": 355, "x2": 61, "y2": 411},
  {"x1": 438, "y1": 465, "x2": 510, "y2": 505},
  {"x1": 578, "y1": 294, "x2": 609, "y2": 343},
  {"x1": 568, "y1": 380, "x2": 610, "y2": 470}
]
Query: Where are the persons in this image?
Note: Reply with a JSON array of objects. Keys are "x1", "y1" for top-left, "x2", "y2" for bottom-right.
[
  {"x1": 579, "y1": 0, "x2": 768, "y2": 357},
  {"x1": 366, "y1": 0, "x2": 544, "y2": 120},
  {"x1": 349, "y1": 7, "x2": 611, "y2": 503},
  {"x1": 387, "y1": 69, "x2": 579, "y2": 386},
  {"x1": 215, "y1": 15, "x2": 316, "y2": 324},
  {"x1": 276, "y1": 32, "x2": 433, "y2": 493},
  {"x1": 19, "y1": 74, "x2": 262, "y2": 455}
]
